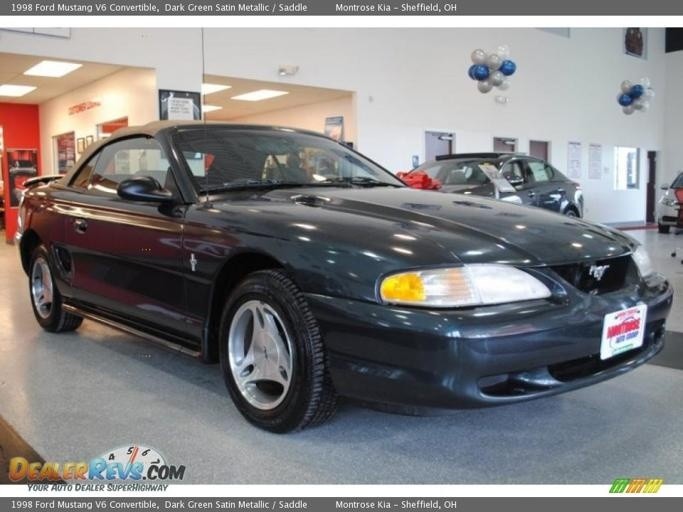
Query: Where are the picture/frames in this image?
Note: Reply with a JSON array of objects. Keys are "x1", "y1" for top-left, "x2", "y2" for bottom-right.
[
  {"x1": 86, "y1": 135, "x2": 93, "y2": 147},
  {"x1": 77, "y1": 138, "x2": 86, "y2": 153}
]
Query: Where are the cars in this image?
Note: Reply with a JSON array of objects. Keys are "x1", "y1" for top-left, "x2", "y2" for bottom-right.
[{"x1": 655, "y1": 172, "x2": 682, "y2": 232}]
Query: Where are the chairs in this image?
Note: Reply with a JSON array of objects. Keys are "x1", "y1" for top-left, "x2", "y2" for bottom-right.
[{"x1": 266, "y1": 166, "x2": 308, "y2": 182}]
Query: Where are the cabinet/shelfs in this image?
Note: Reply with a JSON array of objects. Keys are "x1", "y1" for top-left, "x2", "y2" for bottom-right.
[{"x1": 3, "y1": 147, "x2": 41, "y2": 244}]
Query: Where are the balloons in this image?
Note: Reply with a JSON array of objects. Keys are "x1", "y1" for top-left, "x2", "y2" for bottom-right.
[
  {"x1": 468, "y1": 44, "x2": 516, "y2": 105},
  {"x1": 618, "y1": 77, "x2": 655, "y2": 115}
]
